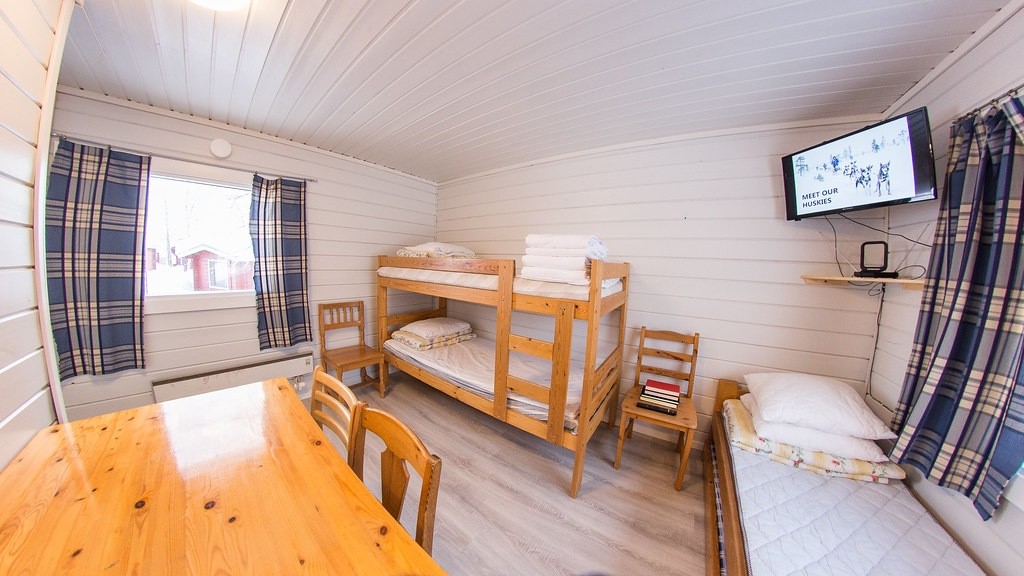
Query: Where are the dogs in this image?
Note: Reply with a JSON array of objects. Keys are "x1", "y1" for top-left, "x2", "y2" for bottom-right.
[
  {"x1": 842, "y1": 161, "x2": 874, "y2": 190},
  {"x1": 873, "y1": 161, "x2": 892, "y2": 197}
]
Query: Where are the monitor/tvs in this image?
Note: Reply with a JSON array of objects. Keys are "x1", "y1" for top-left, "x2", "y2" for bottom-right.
[{"x1": 781, "y1": 106, "x2": 939, "y2": 221}]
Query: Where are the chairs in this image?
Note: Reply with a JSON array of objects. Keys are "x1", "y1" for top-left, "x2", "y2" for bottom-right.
[
  {"x1": 351, "y1": 402, "x2": 442, "y2": 558},
  {"x1": 613, "y1": 326, "x2": 700, "y2": 491},
  {"x1": 317, "y1": 301, "x2": 385, "y2": 406},
  {"x1": 310, "y1": 365, "x2": 367, "y2": 465}
]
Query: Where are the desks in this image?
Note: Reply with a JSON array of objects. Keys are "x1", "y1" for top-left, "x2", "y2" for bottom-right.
[{"x1": 0, "y1": 377, "x2": 450, "y2": 576}]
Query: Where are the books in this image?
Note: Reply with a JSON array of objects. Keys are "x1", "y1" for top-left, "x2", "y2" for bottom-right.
[{"x1": 636, "y1": 379, "x2": 680, "y2": 417}]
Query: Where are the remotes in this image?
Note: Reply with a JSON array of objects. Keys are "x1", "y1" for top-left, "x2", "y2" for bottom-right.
[{"x1": 636, "y1": 402, "x2": 678, "y2": 416}]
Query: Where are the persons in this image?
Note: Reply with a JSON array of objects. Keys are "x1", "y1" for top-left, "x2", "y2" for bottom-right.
[{"x1": 832, "y1": 156, "x2": 840, "y2": 172}]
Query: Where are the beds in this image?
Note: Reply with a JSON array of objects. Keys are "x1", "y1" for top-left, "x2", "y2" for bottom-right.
[
  {"x1": 701, "y1": 378, "x2": 987, "y2": 576},
  {"x1": 376, "y1": 254, "x2": 631, "y2": 499}
]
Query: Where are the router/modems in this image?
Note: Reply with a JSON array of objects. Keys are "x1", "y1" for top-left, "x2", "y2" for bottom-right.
[{"x1": 818, "y1": 223, "x2": 930, "y2": 276}]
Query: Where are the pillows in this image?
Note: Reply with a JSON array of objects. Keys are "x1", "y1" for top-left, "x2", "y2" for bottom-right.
[
  {"x1": 416, "y1": 241, "x2": 475, "y2": 257},
  {"x1": 740, "y1": 392, "x2": 890, "y2": 463},
  {"x1": 745, "y1": 370, "x2": 900, "y2": 440},
  {"x1": 401, "y1": 317, "x2": 471, "y2": 339}
]
